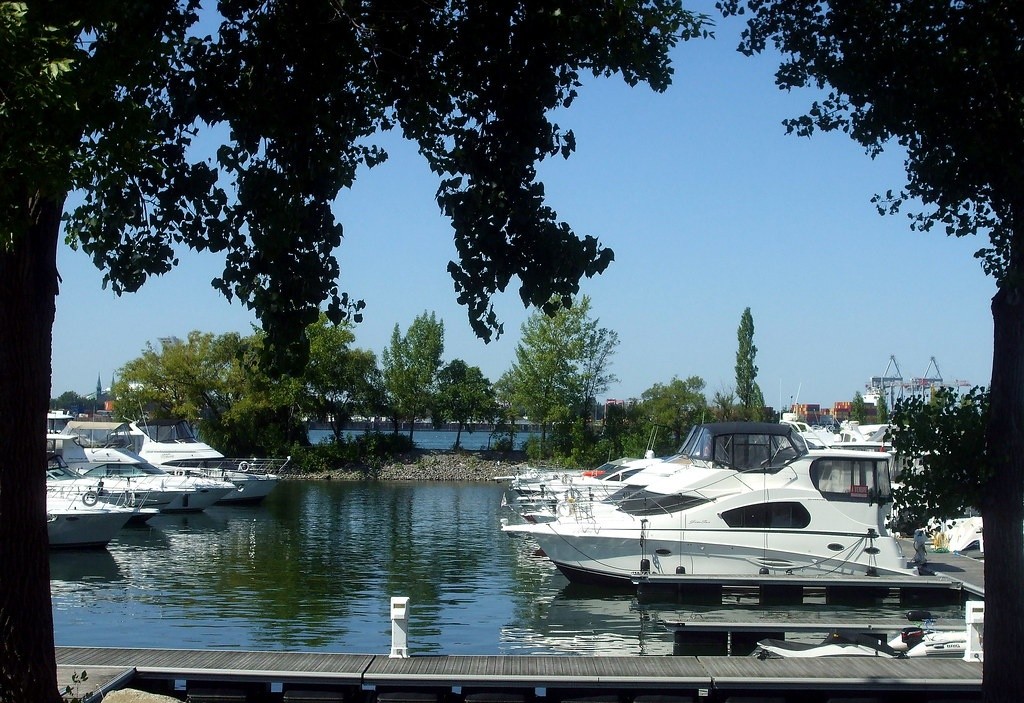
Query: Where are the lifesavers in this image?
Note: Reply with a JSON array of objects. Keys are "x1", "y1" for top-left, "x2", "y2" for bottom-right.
[
  {"x1": 81, "y1": 491, "x2": 99, "y2": 507},
  {"x1": 175, "y1": 468, "x2": 185, "y2": 476},
  {"x1": 561, "y1": 474, "x2": 573, "y2": 486},
  {"x1": 239, "y1": 461, "x2": 249, "y2": 472},
  {"x1": 564, "y1": 489, "x2": 580, "y2": 502},
  {"x1": 556, "y1": 501, "x2": 572, "y2": 517}
]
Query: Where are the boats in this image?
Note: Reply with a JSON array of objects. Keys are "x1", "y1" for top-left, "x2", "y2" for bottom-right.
[
  {"x1": 44, "y1": 415, "x2": 293, "y2": 555},
  {"x1": 495, "y1": 381, "x2": 984, "y2": 589},
  {"x1": 751, "y1": 598, "x2": 985, "y2": 663}
]
